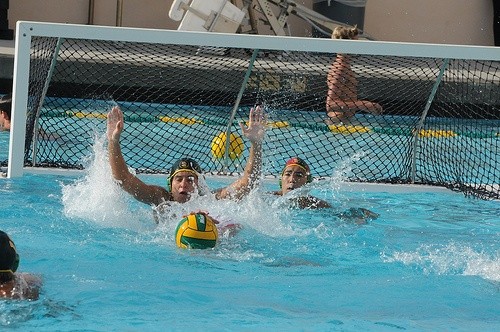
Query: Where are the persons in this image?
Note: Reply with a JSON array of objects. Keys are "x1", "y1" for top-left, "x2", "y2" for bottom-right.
[
  {"x1": 0, "y1": 95, "x2": 11, "y2": 131},
  {"x1": 326, "y1": 24, "x2": 383, "y2": 125},
  {"x1": 0, "y1": 230, "x2": 40, "y2": 300},
  {"x1": 107, "y1": 106, "x2": 268, "y2": 224},
  {"x1": 272, "y1": 156, "x2": 331, "y2": 208}
]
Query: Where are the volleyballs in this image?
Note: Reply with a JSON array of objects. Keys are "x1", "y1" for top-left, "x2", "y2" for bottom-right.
[
  {"x1": 174, "y1": 213, "x2": 218, "y2": 250},
  {"x1": 210, "y1": 131, "x2": 244, "y2": 159}
]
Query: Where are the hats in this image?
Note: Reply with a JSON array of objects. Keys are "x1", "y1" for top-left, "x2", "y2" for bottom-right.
[
  {"x1": 167, "y1": 158, "x2": 203, "y2": 187},
  {"x1": 279, "y1": 157, "x2": 310, "y2": 182},
  {"x1": 0, "y1": 230, "x2": 20, "y2": 283}
]
